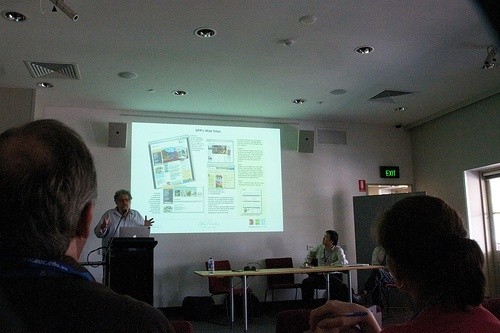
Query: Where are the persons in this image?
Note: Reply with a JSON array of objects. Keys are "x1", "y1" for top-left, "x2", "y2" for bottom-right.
[
  {"x1": 93, "y1": 189, "x2": 155, "y2": 263},
  {"x1": 307, "y1": 194, "x2": 500, "y2": 333},
  {"x1": 351, "y1": 242, "x2": 397, "y2": 310},
  {"x1": 303, "y1": 229, "x2": 349, "y2": 309},
  {"x1": 0, "y1": 117, "x2": 175, "y2": 333}
]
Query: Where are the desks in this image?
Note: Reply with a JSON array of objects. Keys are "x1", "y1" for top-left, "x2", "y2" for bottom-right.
[{"x1": 194, "y1": 264, "x2": 390, "y2": 333}]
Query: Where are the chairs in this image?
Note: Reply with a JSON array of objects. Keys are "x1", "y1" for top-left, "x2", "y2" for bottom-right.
[
  {"x1": 263, "y1": 258, "x2": 305, "y2": 317},
  {"x1": 205, "y1": 260, "x2": 254, "y2": 326}
]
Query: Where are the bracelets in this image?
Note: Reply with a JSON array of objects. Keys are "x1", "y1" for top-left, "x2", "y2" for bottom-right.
[{"x1": 305, "y1": 262, "x2": 309, "y2": 264}]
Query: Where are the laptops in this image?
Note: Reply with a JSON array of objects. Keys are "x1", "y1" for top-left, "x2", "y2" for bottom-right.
[{"x1": 119, "y1": 227, "x2": 150, "y2": 238}]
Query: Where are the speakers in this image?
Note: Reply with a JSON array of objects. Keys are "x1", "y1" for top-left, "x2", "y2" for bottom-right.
[
  {"x1": 108, "y1": 122, "x2": 127, "y2": 147},
  {"x1": 297, "y1": 130, "x2": 314, "y2": 153}
]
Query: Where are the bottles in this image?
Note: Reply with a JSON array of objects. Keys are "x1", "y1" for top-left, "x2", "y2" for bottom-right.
[{"x1": 208, "y1": 257, "x2": 214, "y2": 272}]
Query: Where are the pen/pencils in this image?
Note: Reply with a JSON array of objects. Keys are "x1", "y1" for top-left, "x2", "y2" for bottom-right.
[{"x1": 324, "y1": 311, "x2": 367, "y2": 317}]
